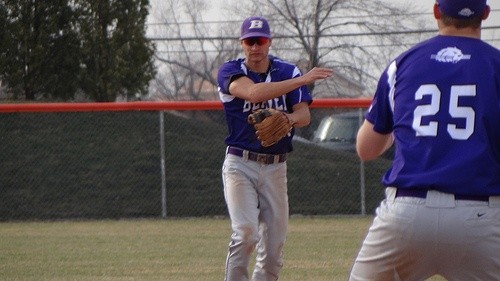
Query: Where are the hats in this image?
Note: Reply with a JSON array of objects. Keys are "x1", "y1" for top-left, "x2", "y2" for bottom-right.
[
  {"x1": 239, "y1": 16, "x2": 270, "y2": 40},
  {"x1": 435, "y1": 0, "x2": 486, "y2": 18}
]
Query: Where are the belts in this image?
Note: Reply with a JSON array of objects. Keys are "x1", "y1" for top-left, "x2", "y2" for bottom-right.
[
  {"x1": 228, "y1": 148, "x2": 286, "y2": 164},
  {"x1": 395, "y1": 187, "x2": 489, "y2": 201}
]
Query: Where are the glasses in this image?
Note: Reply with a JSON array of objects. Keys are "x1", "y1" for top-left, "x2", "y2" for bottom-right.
[{"x1": 243, "y1": 37, "x2": 268, "y2": 46}]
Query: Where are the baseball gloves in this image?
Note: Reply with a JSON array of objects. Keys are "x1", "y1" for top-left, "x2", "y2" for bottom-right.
[{"x1": 248, "y1": 107, "x2": 294, "y2": 147}]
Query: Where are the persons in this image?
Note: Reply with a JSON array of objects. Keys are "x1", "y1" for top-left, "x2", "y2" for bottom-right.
[
  {"x1": 348, "y1": 0, "x2": 500, "y2": 281},
  {"x1": 218, "y1": 16, "x2": 334, "y2": 281}
]
